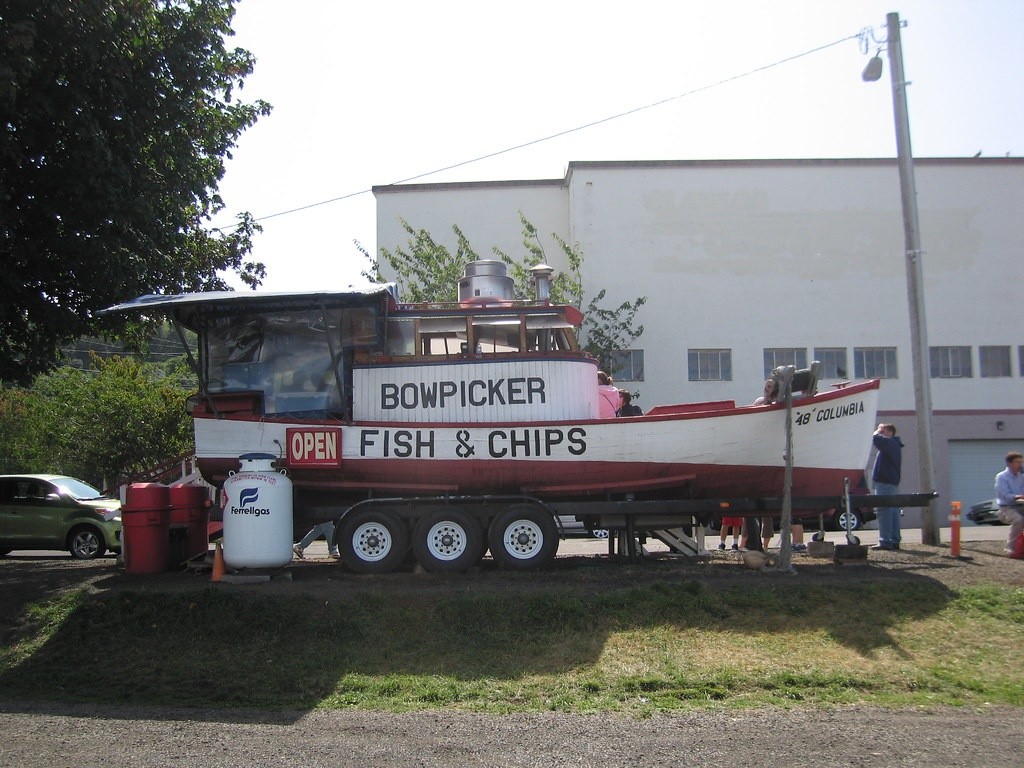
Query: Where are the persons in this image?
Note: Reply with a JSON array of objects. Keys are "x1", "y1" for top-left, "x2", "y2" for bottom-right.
[
  {"x1": 310, "y1": 373, "x2": 334, "y2": 392},
  {"x1": 293, "y1": 521, "x2": 339, "y2": 560},
  {"x1": 717, "y1": 515, "x2": 807, "y2": 554},
  {"x1": 598, "y1": 371, "x2": 620, "y2": 419},
  {"x1": 616, "y1": 388, "x2": 642, "y2": 417},
  {"x1": 292, "y1": 371, "x2": 308, "y2": 392},
  {"x1": 995, "y1": 452, "x2": 1024, "y2": 554},
  {"x1": 873, "y1": 422, "x2": 905, "y2": 547}
]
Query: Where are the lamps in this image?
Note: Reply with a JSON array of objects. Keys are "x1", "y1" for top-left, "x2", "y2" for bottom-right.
[{"x1": 996, "y1": 420, "x2": 1004, "y2": 430}]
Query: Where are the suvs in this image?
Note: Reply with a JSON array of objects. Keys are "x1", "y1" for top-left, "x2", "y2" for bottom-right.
[
  {"x1": 707, "y1": 473, "x2": 876, "y2": 534},
  {"x1": 0, "y1": 473, "x2": 122, "y2": 560}
]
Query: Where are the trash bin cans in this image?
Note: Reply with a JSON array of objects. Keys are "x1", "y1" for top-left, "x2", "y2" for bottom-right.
[
  {"x1": 119, "y1": 483, "x2": 173, "y2": 576},
  {"x1": 169, "y1": 484, "x2": 213, "y2": 561}
]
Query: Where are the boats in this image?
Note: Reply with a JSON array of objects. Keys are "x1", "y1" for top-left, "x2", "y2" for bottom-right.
[{"x1": 93, "y1": 258, "x2": 888, "y2": 503}]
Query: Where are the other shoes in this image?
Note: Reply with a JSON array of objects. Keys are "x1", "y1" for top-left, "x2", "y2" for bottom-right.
[
  {"x1": 719, "y1": 543, "x2": 726, "y2": 550},
  {"x1": 732, "y1": 544, "x2": 738, "y2": 550},
  {"x1": 793, "y1": 544, "x2": 807, "y2": 552},
  {"x1": 872, "y1": 545, "x2": 899, "y2": 550}
]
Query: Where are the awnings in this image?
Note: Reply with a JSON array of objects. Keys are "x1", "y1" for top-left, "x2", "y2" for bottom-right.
[{"x1": 100, "y1": 280, "x2": 388, "y2": 421}]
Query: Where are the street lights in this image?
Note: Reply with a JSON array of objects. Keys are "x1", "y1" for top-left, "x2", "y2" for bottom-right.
[{"x1": 862, "y1": 47, "x2": 945, "y2": 547}]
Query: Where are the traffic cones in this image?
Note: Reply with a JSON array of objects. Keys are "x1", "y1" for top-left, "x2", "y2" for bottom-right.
[{"x1": 210, "y1": 539, "x2": 226, "y2": 584}]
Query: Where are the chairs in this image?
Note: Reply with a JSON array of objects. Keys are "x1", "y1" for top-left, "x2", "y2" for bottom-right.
[{"x1": 26, "y1": 483, "x2": 40, "y2": 499}]
